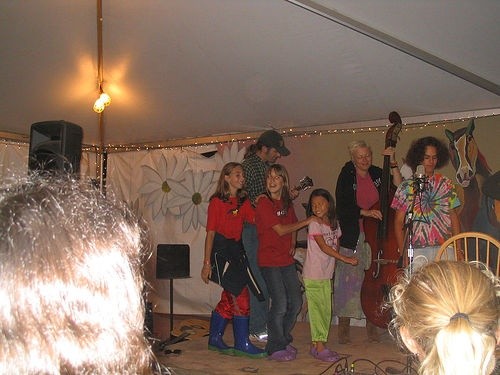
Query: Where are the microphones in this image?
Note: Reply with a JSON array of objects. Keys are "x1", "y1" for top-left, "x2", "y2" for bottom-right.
[{"x1": 416, "y1": 165, "x2": 425, "y2": 198}]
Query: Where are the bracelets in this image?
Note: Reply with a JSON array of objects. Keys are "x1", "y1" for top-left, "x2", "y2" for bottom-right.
[{"x1": 391, "y1": 164, "x2": 398, "y2": 169}]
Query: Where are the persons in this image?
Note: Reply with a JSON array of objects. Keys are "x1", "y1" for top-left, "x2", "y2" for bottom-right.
[
  {"x1": 239, "y1": 131, "x2": 300, "y2": 350},
  {"x1": 333, "y1": 140, "x2": 403, "y2": 344},
  {"x1": 302, "y1": 188, "x2": 358, "y2": 363},
  {"x1": 201, "y1": 162, "x2": 267, "y2": 358},
  {"x1": 0, "y1": 173, "x2": 160, "y2": 375},
  {"x1": 391, "y1": 137, "x2": 462, "y2": 278},
  {"x1": 389, "y1": 255, "x2": 500, "y2": 375},
  {"x1": 255, "y1": 164, "x2": 323, "y2": 362}
]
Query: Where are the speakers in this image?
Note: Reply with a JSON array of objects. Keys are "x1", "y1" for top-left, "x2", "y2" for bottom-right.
[{"x1": 27, "y1": 120, "x2": 81, "y2": 181}]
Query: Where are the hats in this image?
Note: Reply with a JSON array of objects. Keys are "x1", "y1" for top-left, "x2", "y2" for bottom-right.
[{"x1": 259, "y1": 130, "x2": 291, "y2": 157}]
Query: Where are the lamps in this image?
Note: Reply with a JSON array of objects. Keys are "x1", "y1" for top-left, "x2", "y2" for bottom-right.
[
  {"x1": 100, "y1": 85, "x2": 111, "y2": 107},
  {"x1": 93, "y1": 98, "x2": 105, "y2": 114}
]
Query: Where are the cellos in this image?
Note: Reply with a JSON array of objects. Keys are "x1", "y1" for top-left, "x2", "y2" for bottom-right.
[{"x1": 359, "y1": 111, "x2": 409, "y2": 332}]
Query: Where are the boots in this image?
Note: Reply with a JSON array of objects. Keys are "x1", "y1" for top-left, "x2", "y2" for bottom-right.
[
  {"x1": 208, "y1": 311, "x2": 235, "y2": 353},
  {"x1": 232, "y1": 316, "x2": 267, "y2": 358}
]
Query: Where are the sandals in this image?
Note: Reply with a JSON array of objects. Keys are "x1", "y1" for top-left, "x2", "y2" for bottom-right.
[
  {"x1": 285, "y1": 344, "x2": 298, "y2": 354},
  {"x1": 310, "y1": 348, "x2": 340, "y2": 362},
  {"x1": 269, "y1": 351, "x2": 296, "y2": 361}
]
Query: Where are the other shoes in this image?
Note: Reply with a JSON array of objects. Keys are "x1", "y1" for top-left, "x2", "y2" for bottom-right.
[
  {"x1": 338, "y1": 325, "x2": 350, "y2": 345},
  {"x1": 367, "y1": 325, "x2": 379, "y2": 344}
]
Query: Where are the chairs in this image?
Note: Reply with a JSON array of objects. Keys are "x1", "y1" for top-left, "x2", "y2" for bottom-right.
[{"x1": 435, "y1": 232, "x2": 500, "y2": 278}]
